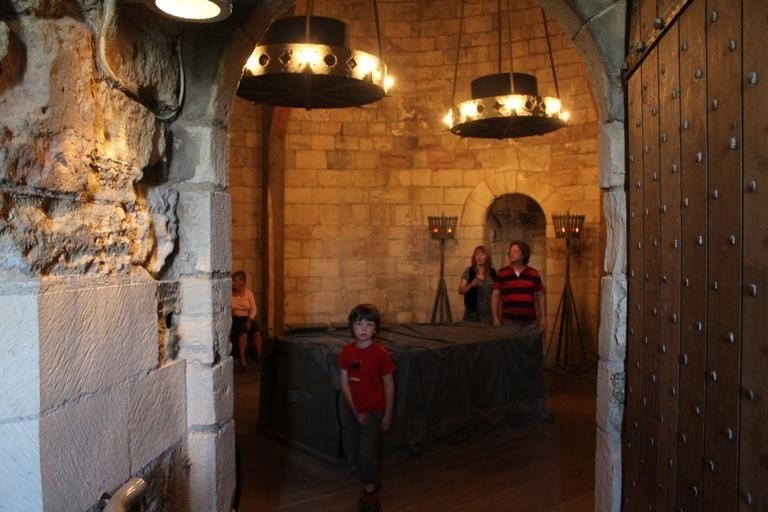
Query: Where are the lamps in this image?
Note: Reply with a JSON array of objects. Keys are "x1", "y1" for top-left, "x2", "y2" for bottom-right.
[
  {"x1": 234, "y1": 0, "x2": 394, "y2": 109},
  {"x1": 441, "y1": 0, "x2": 571, "y2": 141},
  {"x1": 143, "y1": 0, "x2": 237, "y2": 29}
]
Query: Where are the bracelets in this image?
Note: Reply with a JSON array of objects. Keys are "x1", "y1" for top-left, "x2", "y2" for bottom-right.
[{"x1": 468, "y1": 283, "x2": 472, "y2": 289}]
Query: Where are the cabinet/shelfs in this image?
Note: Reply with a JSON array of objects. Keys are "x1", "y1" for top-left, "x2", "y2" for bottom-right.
[{"x1": 253, "y1": 321, "x2": 551, "y2": 473}]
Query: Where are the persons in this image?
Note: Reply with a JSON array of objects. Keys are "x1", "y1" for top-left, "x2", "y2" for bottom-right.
[
  {"x1": 459, "y1": 246, "x2": 497, "y2": 324},
  {"x1": 490, "y1": 240, "x2": 547, "y2": 338},
  {"x1": 336, "y1": 303, "x2": 396, "y2": 511},
  {"x1": 231, "y1": 272, "x2": 263, "y2": 370}
]
